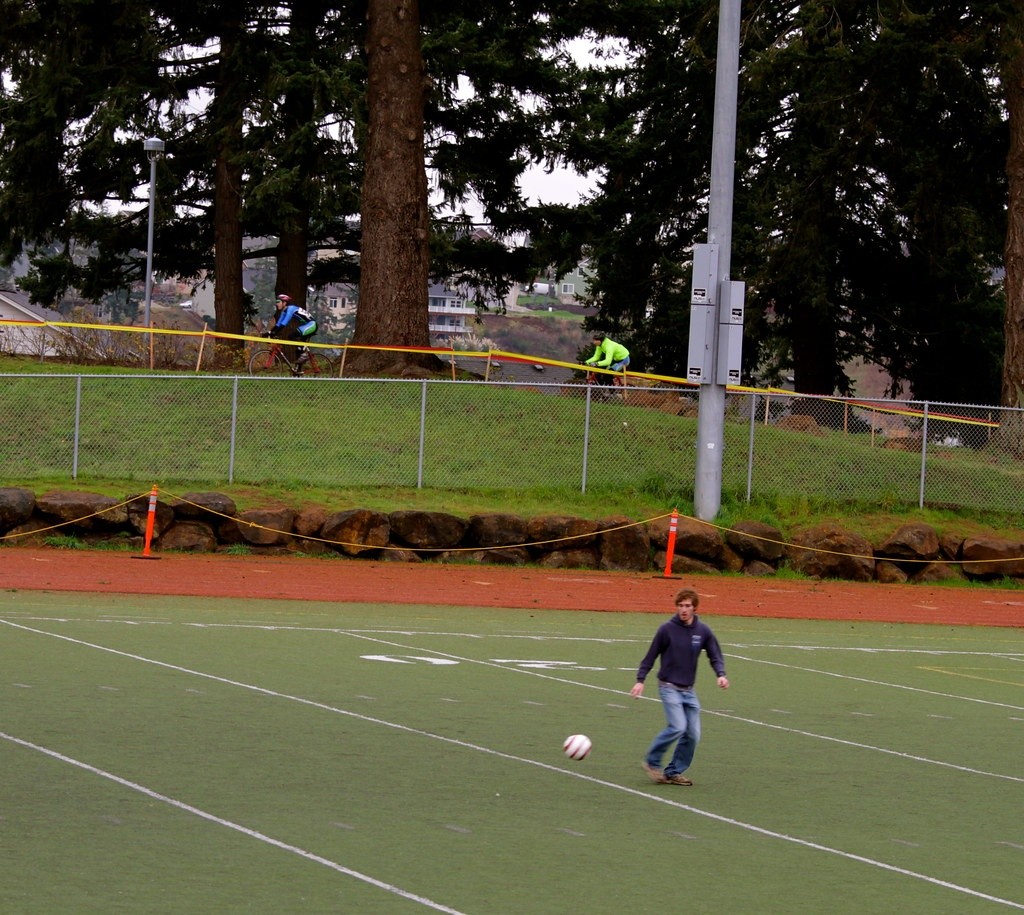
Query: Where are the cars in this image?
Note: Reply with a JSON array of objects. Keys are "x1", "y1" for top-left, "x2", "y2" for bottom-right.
[
  {"x1": 931, "y1": 430, "x2": 964, "y2": 448},
  {"x1": 180, "y1": 301, "x2": 192, "y2": 308}
]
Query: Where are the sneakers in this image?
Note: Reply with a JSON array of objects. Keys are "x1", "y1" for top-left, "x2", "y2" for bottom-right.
[
  {"x1": 664, "y1": 774, "x2": 693, "y2": 786},
  {"x1": 295, "y1": 353, "x2": 309, "y2": 364},
  {"x1": 641, "y1": 762, "x2": 664, "y2": 783}
]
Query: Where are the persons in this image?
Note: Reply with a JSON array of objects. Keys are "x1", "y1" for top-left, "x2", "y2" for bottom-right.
[
  {"x1": 584, "y1": 332, "x2": 630, "y2": 399},
  {"x1": 629, "y1": 590, "x2": 730, "y2": 786},
  {"x1": 262, "y1": 294, "x2": 318, "y2": 374}
]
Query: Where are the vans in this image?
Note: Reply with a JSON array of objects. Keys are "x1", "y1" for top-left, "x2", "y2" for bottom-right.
[{"x1": 527, "y1": 283, "x2": 549, "y2": 296}]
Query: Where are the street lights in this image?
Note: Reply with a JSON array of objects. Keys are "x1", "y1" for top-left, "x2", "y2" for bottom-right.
[{"x1": 143, "y1": 137, "x2": 165, "y2": 348}]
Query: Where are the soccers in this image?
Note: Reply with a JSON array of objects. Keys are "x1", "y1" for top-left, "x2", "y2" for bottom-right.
[{"x1": 562, "y1": 734, "x2": 592, "y2": 761}]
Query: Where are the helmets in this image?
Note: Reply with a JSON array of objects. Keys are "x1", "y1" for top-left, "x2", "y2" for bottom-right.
[
  {"x1": 275, "y1": 294, "x2": 292, "y2": 301},
  {"x1": 592, "y1": 331, "x2": 605, "y2": 339}
]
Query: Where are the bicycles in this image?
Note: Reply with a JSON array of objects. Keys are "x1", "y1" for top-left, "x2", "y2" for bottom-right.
[
  {"x1": 577, "y1": 361, "x2": 639, "y2": 404},
  {"x1": 248, "y1": 332, "x2": 334, "y2": 378}
]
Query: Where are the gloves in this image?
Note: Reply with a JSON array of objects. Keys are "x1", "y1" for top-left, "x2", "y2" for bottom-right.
[{"x1": 589, "y1": 362, "x2": 597, "y2": 367}]
